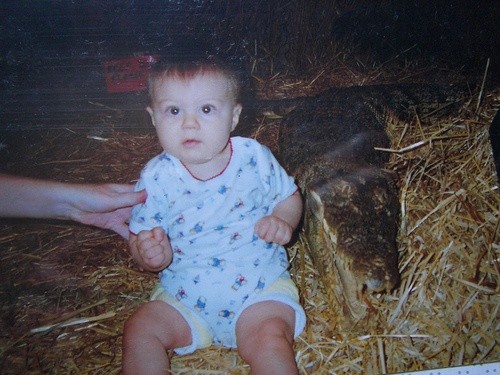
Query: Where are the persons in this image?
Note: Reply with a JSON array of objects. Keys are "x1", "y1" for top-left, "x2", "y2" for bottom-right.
[
  {"x1": 1, "y1": 175, "x2": 148, "y2": 240},
  {"x1": 120, "y1": 38, "x2": 309, "y2": 375}
]
